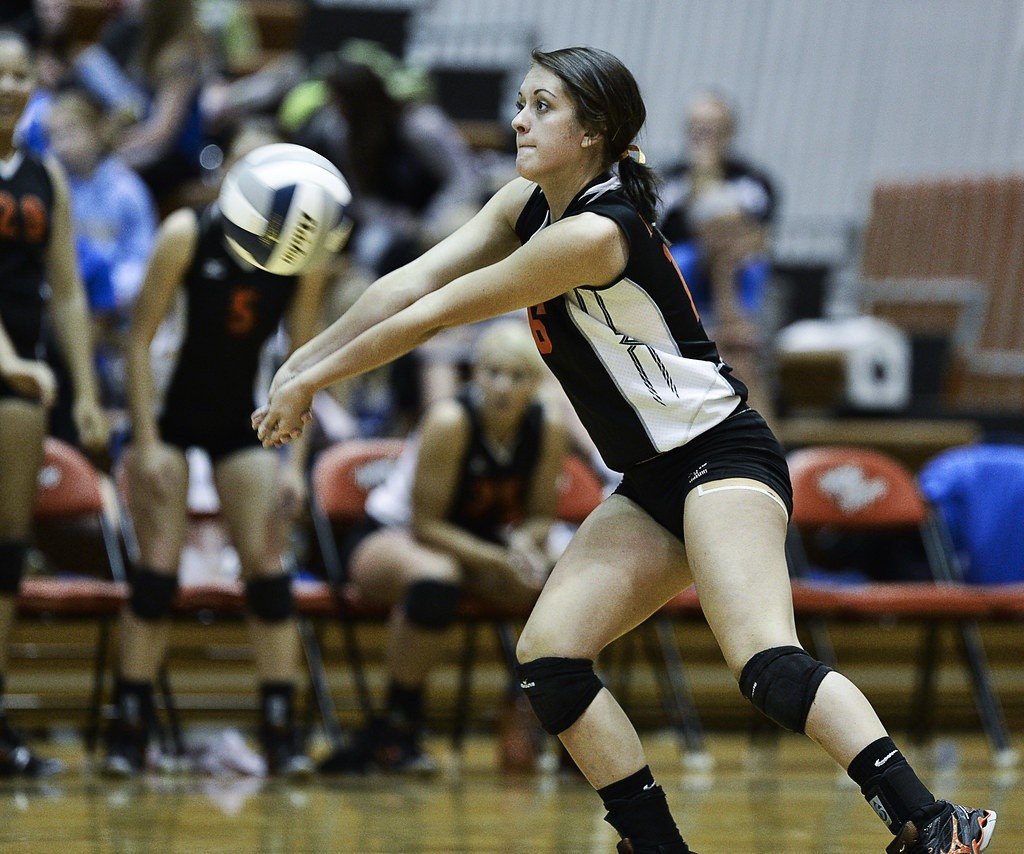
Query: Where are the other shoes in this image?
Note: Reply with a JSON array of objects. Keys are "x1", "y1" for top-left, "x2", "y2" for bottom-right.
[
  {"x1": 268, "y1": 751, "x2": 314, "y2": 776},
  {"x1": 1, "y1": 735, "x2": 38, "y2": 776},
  {"x1": 105, "y1": 740, "x2": 140, "y2": 774},
  {"x1": 370, "y1": 726, "x2": 414, "y2": 768}
]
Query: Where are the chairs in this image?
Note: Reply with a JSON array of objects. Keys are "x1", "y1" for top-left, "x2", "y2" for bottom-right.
[
  {"x1": 313, "y1": 436, "x2": 558, "y2": 774},
  {"x1": 783, "y1": 444, "x2": 1018, "y2": 767},
  {"x1": 456, "y1": 453, "x2": 708, "y2": 764},
  {"x1": 86, "y1": 445, "x2": 340, "y2": 761},
  {"x1": 909, "y1": 445, "x2": 1024, "y2": 749},
  {"x1": 18, "y1": 437, "x2": 169, "y2": 754}
]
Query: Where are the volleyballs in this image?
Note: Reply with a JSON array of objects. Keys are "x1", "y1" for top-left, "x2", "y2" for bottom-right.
[{"x1": 216, "y1": 140, "x2": 360, "y2": 280}]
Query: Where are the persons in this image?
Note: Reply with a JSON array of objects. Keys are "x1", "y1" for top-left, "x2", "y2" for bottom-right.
[
  {"x1": 1, "y1": 1, "x2": 778, "y2": 784},
  {"x1": 247, "y1": 45, "x2": 1001, "y2": 854}
]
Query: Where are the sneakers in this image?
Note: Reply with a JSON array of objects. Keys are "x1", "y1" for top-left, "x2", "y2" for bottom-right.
[{"x1": 882, "y1": 800, "x2": 997, "y2": 854}]
